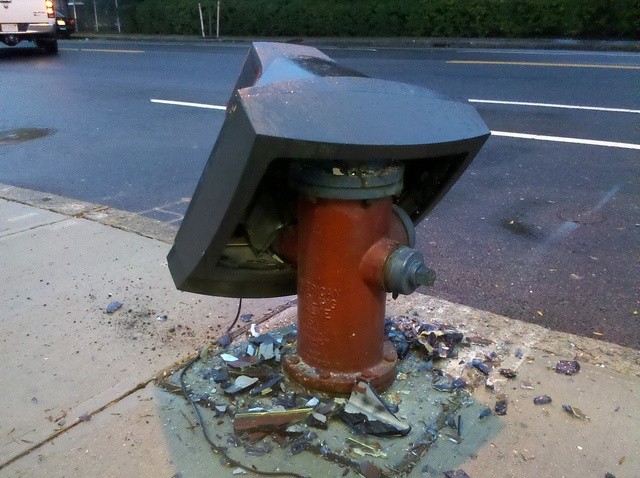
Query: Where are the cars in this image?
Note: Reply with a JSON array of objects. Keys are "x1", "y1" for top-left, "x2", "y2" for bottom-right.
[{"x1": 55, "y1": 10, "x2": 73, "y2": 35}]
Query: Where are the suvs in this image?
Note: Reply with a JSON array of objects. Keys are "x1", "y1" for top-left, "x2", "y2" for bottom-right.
[{"x1": 0, "y1": 0, "x2": 58, "y2": 55}]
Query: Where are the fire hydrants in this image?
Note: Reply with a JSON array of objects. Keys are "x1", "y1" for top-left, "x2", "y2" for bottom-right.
[{"x1": 247, "y1": 160, "x2": 437, "y2": 393}]
